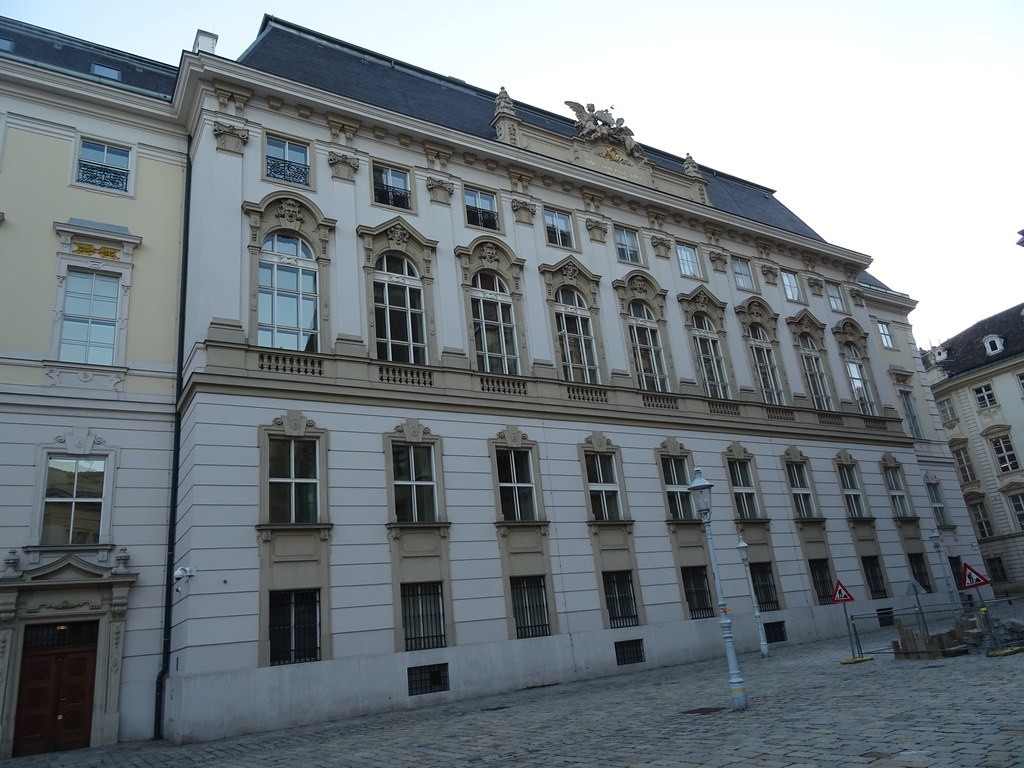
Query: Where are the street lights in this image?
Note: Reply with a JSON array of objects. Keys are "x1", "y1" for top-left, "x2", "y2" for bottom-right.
[
  {"x1": 928, "y1": 528, "x2": 962, "y2": 625},
  {"x1": 685, "y1": 464, "x2": 749, "y2": 713},
  {"x1": 735, "y1": 532, "x2": 771, "y2": 659}
]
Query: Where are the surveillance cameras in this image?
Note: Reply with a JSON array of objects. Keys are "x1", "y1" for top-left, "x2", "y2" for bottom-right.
[{"x1": 174, "y1": 571, "x2": 184, "y2": 580}]
